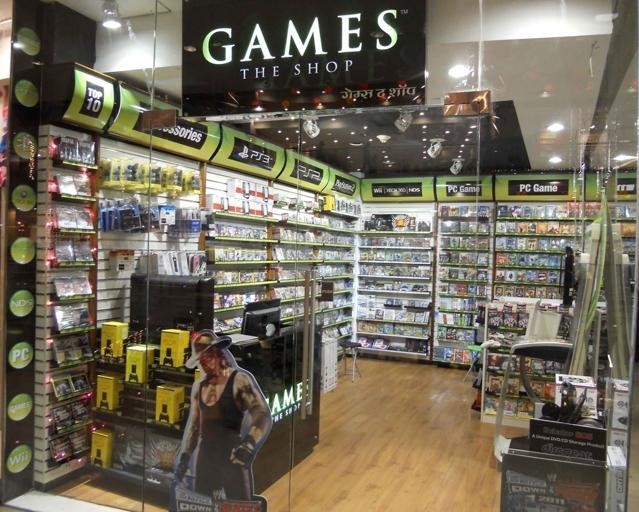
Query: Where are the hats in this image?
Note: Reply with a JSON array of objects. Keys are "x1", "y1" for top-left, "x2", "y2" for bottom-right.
[{"x1": 185, "y1": 331, "x2": 232, "y2": 369}]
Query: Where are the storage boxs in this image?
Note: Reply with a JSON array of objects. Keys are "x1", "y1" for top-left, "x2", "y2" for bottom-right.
[
  {"x1": 500, "y1": 374, "x2": 606, "y2": 512},
  {"x1": 606, "y1": 378, "x2": 631, "y2": 512}
]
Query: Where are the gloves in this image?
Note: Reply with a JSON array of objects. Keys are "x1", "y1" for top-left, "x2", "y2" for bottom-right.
[
  {"x1": 175, "y1": 452, "x2": 191, "y2": 482},
  {"x1": 233, "y1": 435, "x2": 257, "y2": 465}
]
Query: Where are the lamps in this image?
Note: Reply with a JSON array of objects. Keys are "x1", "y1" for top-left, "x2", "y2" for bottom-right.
[{"x1": 101, "y1": 0, "x2": 122, "y2": 30}]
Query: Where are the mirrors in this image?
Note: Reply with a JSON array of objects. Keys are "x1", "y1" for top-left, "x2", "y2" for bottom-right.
[{"x1": 583, "y1": 0, "x2": 639, "y2": 446}]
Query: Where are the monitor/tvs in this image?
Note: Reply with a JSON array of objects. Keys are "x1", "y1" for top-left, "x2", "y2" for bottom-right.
[
  {"x1": 241, "y1": 298, "x2": 281, "y2": 341},
  {"x1": 563, "y1": 246, "x2": 574, "y2": 307},
  {"x1": 130, "y1": 273, "x2": 214, "y2": 346}
]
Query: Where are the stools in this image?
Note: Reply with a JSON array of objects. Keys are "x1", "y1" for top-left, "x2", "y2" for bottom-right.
[
  {"x1": 336, "y1": 340, "x2": 363, "y2": 382},
  {"x1": 461, "y1": 344, "x2": 484, "y2": 384}
]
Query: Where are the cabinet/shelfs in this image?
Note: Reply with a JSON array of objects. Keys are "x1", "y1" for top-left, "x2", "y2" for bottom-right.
[
  {"x1": 356, "y1": 170, "x2": 581, "y2": 366},
  {"x1": 585, "y1": 170, "x2": 639, "y2": 376},
  {"x1": 49, "y1": 125, "x2": 362, "y2": 488}
]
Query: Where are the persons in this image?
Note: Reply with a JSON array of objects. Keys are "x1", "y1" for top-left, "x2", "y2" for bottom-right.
[{"x1": 167, "y1": 329, "x2": 275, "y2": 504}]
